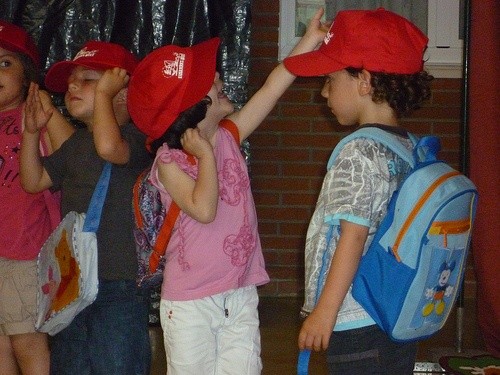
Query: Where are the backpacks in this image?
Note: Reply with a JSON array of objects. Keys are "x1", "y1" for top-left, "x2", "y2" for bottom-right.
[
  {"x1": 326, "y1": 127, "x2": 478, "y2": 343},
  {"x1": 129, "y1": 119, "x2": 240, "y2": 290}
]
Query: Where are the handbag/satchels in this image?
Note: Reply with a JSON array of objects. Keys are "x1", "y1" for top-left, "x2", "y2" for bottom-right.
[{"x1": 35, "y1": 211, "x2": 99, "y2": 336}]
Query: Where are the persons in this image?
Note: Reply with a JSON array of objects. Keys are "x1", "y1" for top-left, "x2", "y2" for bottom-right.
[
  {"x1": 0, "y1": 23, "x2": 81, "y2": 375},
  {"x1": 18, "y1": 41, "x2": 153, "y2": 375},
  {"x1": 282, "y1": 7, "x2": 434, "y2": 375},
  {"x1": 127, "y1": 7, "x2": 336, "y2": 375}
]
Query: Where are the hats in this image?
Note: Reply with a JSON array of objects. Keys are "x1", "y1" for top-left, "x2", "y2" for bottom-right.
[
  {"x1": 127, "y1": 37, "x2": 222, "y2": 152},
  {"x1": 45, "y1": 42, "x2": 139, "y2": 92},
  {"x1": 283, "y1": 8, "x2": 430, "y2": 75},
  {"x1": 0, "y1": 19, "x2": 40, "y2": 80}
]
